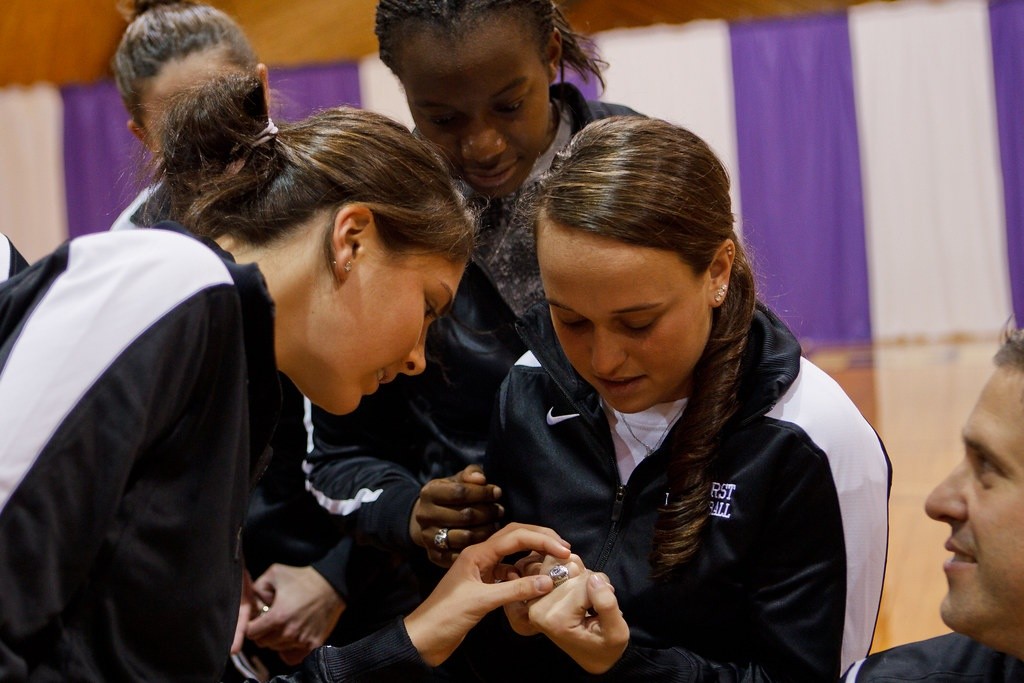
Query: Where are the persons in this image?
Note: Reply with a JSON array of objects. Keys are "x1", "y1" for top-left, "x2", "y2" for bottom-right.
[
  {"x1": 489, "y1": 112, "x2": 893, "y2": 683},
  {"x1": 836, "y1": 318, "x2": 1024, "y2": 683},
  {"x1": 106, "y1": 0, "x2": 650, "y2": 683},
  {"x1": 0, "y1": 76, "x2": 572, "y2": 683}
]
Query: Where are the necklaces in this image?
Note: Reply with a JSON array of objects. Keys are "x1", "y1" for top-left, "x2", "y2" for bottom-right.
[{"x1": 618, "y1": 398, "x2": 687, "y2": 457}]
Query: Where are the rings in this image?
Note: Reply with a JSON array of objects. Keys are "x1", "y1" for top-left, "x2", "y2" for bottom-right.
[
  {"x1": 435, "y1": 528, "x2": 448, "y2": 550},
  {"x1": 548, "y1": 565, "x2": 570, "y2": 587}
]
